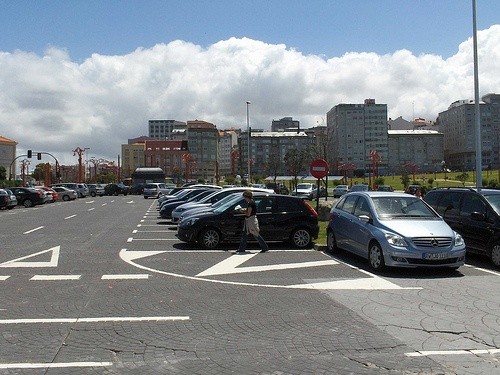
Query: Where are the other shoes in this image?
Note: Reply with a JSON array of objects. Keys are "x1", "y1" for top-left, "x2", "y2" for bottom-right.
[
  {"x1": 235, "y1": 248, "x2": 245, "y2": 254},
  {"x1": 260, "y1": 247, "x2": 268, "y2": 253}
]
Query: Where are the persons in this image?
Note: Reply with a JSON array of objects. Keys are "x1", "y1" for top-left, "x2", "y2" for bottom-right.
[
  {"x1": 236, "y1": 190, "x2": 270, "y2": 253},
  {"x1": 249, "y1": 183, "x2": 253, "y2": 187},
  {"x1": 407, "y1": 186, "x2": 427, "y2": 199},
  {"x1": 280, "y1": 185, "x2": 289, "y2": 195}
]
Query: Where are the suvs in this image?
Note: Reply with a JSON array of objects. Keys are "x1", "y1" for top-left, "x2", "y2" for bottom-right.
[
  {"x1": 158, "y1": 184, "x2": 320, "y2": 249},
  {"x1": 142, "y1": 182, "x2": 170, "y2": 199}
]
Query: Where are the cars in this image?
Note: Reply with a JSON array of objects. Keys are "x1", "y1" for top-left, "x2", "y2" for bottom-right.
[
  {"x1": 0, "y1": 182, "x2": 129, "y2": 210},
  {"x1": 349, "y1": 184, "x2": 372, "y2": 191},
  {"x1": 404, "y1": 184, "x2": 421, "y2": 195},
  {"x1": 325, "y1": 191, "x2": 466, "y2": 272},
  {"x1": 422, "y1": 186, "x2": 500, "y2": 268},
  {"x1": 333, "y1": 184, "x2": 350, "y2": 198},
  {"x1": 292, "y1": 182, "x2": 318, "y2": 200}
]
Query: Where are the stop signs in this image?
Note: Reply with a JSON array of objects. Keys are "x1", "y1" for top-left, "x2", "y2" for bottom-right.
[{"x1": 310, "y1": 160, "x2": 329, "y2": 179}]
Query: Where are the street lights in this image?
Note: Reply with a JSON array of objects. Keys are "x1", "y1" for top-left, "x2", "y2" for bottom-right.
[
  {"x1": 165, "y1": 137, "x2": 168, "y2": 183},
  {"x1": 246, "y1": 100, "x2": 251, "y2": 186}
]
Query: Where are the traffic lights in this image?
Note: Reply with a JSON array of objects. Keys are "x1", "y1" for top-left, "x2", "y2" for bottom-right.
[{"x1": 38, "y1": 153, "x2": 41, "y2": 160}]
